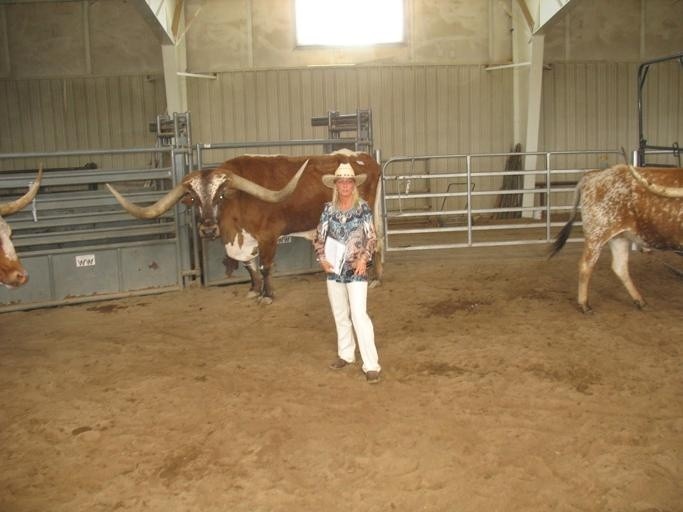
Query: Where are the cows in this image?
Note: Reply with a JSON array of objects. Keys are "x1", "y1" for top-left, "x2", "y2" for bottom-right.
[
  {"x1": 105, "y1": 148, "x2": 385, "y2": 308},
  {"x1": 544, "y1": 145, "x2": 683, "y2": 313},
  {"x1": 1, "y1": 162, "x2": 44, "y2": 288}
]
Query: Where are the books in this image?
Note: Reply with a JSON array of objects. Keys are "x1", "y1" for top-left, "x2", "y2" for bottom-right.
[{"x1": 323, "y1": 235, "x2": 348, "y2": 276}]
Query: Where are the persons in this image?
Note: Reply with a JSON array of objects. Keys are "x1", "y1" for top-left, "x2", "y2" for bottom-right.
[{"x1": 311, "y1": 162, "x2": 381, "y2": 383}]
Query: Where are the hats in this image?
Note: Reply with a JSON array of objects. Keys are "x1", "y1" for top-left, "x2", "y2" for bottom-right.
[{"x1": 321, "y1": 162, "x2": 368, "y2": 190}]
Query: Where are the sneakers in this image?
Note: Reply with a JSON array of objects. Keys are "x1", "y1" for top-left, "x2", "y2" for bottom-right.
[
  {"x1": 366, "y1": 371, "x2": 380, "y2": 384},
  {"x1": 329, "y1": 357, "x2": 349, "y2": 370}
]
